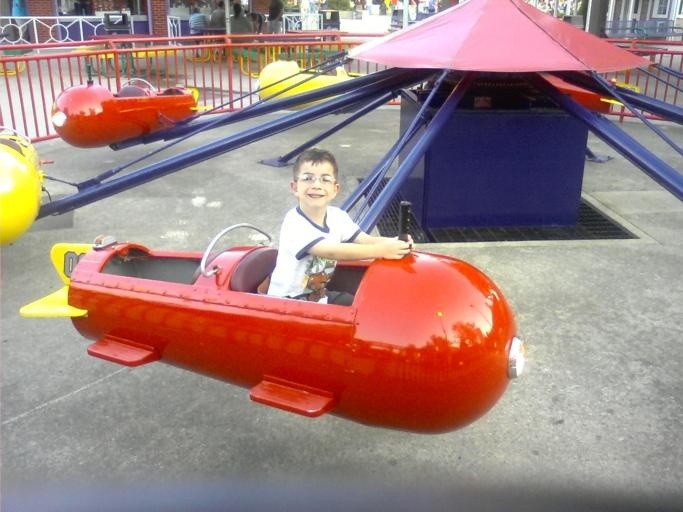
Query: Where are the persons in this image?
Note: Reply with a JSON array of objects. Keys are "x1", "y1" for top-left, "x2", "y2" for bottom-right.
[
  {"x1": 536, "y1": 0, "x2": 578, "y2": 16},
  {"x1": 246, "y1": 11, "x2": 265, "y2": 35},
  {"x1": 241, "y1": 5, "x2": 245, "y2": 16},
  {"x1": 354, "y1": 0, "x2": 436, "y2": 26},
  {"x1": 266, "y1": 148, "x2": 416, "y2": 306},
  {"x1": 210, "y1": 0, "x2": 225, "y2": 28},
  {"x1": 11, "y1": 0, "x2": 29, "y2": 45},
  {"x1": 229, "y1": 3, "x2": 254, "y2": 49},
  {"x1": 189, "y1": 8, "x2": 208, "y2": 58},
  {"x1": 267, "y1": 0, "x2": 284, "y2": 34}
]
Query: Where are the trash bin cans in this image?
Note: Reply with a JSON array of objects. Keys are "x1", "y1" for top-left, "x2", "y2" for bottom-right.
[
  {"x1": 319, "y1": 10, "x2": 340, "y2": 31},
  {"x1": 564, "y1": 16, "x2": 584, "y2": 31},
  {"x1": 104, "y1": 12, "x2": 131, "y2": 35},
  {"x1": 392, "y1": 9, "x2": 411, "y2": 25}
]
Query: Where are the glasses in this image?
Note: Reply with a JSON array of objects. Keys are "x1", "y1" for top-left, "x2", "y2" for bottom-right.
[{"x1": 298, "y1": 175, "x2": 336, "y2": 184}]
